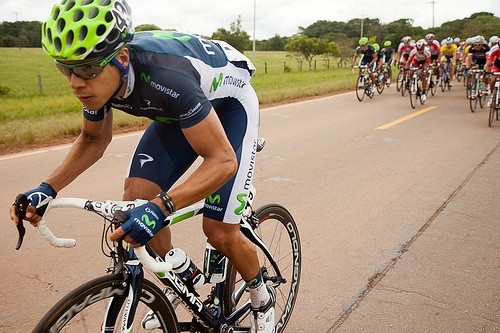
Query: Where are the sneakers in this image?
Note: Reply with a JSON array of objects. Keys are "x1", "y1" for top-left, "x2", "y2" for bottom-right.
[
  {"x1": 250, "y1": 284, "x2": 276, "y2": 333},
  {"x1": 140, "y1": 287, "x2": 181, "y2": 330}
]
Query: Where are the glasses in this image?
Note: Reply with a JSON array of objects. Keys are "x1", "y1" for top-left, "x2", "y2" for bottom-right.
[{"x1": 54, "y1": 41, "x2": 128, "y2": 80}]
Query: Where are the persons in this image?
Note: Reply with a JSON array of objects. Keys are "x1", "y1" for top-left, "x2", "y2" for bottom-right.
[
  {"x1": 406, "y1": 39, "x2": 431, "y2": 101},
  {"x1": 394, "y1": 36, "x2": 415, "y2": 83},
  {"x1": 372, "y1": 44, "x2": 384, "y2": 82},
  {"x1": 381, "y1": 41, "x2": 394, "y2": 84},
  {"x1": 350, "y1": 37, "x2": 377, "y2": 92},
  {"x1": 0, "y1": 0, "x2": 277, "y2": 333},
  {"x1": 425, "y1": 33, "x2": 440, "y2": 79},
  {"x1": 440, "y1": 35, "x2": 500, "y2": 107}
]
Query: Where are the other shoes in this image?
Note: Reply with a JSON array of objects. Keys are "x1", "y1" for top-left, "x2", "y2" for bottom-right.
[
  {"x1": 421, "y1": 94, "x2": 426, "y2": 101},
  {"x1": 482, "y1": 91, "x2": 488, "y2": 96},
  {"x1": 378, "y1": 77, "x2": 382, "y2": 82},
  {"x1": 405, "y1": 82, "x2": 408, "y2": 89},
  {"x1": 487, "y1": 98, "x2": 492, "y2": 107},
  {"x1": 386, "y1": 78, "x2": 390, "y2": 84},
  {"x1": 372, "y1": 86, "x2": 377, "y2": 92},
  {"x1": 449, "y1": 81, "x2": 452, "y2": 87},
  {"x1": 472, "y1": 90, "x2": 475, "y2": 100},
  {"x1": 359, "y1": 83, "x2": 362, "y2": 88}
]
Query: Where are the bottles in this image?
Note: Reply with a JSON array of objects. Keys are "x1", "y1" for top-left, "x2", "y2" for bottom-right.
[
  {"x1": 202, "y1": 236, "x2": 228, "y2": 284},
  {"x1": 164, "y1": 248, "x2": 205, "y2": 289}
]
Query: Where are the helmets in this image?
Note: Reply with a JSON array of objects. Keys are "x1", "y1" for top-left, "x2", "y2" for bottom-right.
[
  {"x1": 400, "y1": 33, "x2": 499, "y2": 46},
  {"x1": 359, "y1": 37, "x2": 368, "y2": 45},
  {"x1": 40, "y1": 0, "x2": 134, "y2": 61},
  {"x1": 372, "y1": 44, "x2": 379, "y2": 51},
  {"x1": 384, "y1": 41, "x2": 391, "y2": 46}
]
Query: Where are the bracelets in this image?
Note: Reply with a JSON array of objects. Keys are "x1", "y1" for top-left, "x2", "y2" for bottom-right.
[{"x1": 156, "y1": 192, "x2": 175, "y2": 215}]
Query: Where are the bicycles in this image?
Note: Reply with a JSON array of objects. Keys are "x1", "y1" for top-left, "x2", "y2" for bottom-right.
[
  {"x1": 350, "y1": 59, "x2": 500, "y2": 128},
  {"x1": 14, "y1": 138, "x2": 302, "y2": 333}
]
